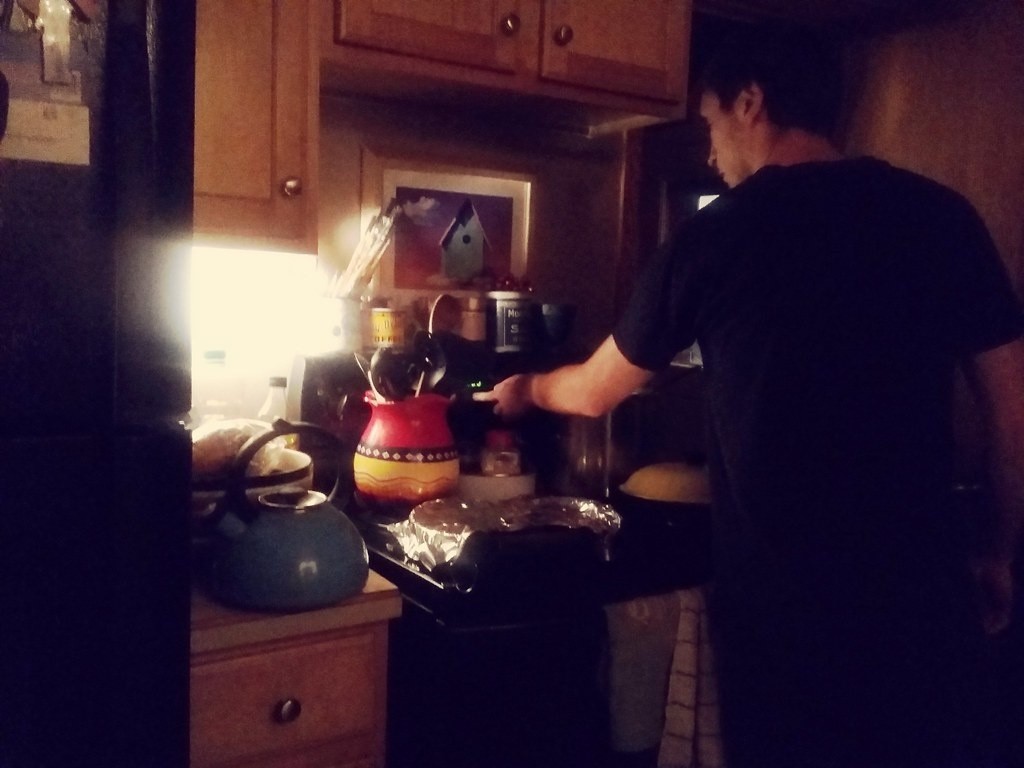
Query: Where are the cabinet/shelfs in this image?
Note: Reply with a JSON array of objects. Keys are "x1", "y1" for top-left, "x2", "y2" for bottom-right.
[
  {"x1": 184, "y1": 617, "x2": 388, "y2": 768},
  {"x1": 186, "y1": 2, "x2": 696, "y2": 255}
]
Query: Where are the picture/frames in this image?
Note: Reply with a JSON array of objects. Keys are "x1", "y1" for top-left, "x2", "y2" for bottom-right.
[{"x1": 361, "y1": 144, "x2": 540, "y2": 310}]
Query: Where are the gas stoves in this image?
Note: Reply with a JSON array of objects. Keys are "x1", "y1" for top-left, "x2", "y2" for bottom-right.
[{"x1": 356, "y1": 489, "x2": 712, "y2": 620}]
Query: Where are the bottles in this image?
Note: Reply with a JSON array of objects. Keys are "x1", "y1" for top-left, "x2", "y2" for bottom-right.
[
  {"x1": 480, "y1": 431, "x2": 520, "y2": 478},
  {"x1": 256, "y1": 375, "x2": 288, "y2": 424},
  {"x1": 460, "y1": 298, "x2": 489, "y2": 341},
  {"x1": 353, "y1": 391, "x2": 460, "y2": 501}
]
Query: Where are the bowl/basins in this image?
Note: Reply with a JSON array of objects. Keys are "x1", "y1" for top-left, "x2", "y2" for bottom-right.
[{"x1": 619, "y1": 462, "x2": 710, "y2": 504}]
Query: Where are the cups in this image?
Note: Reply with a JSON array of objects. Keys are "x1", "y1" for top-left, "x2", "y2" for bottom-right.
[
  {"x1": 370, "y1": 308, "x2": 407, "y2": 349},
  {"x1": 486, "y1": 291, "x2": 533, "y2": 353}
]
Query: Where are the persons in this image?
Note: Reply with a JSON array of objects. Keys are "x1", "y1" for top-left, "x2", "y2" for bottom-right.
[{"x1": 471, "y1": 13, "x2": 1024, "y2": 768}]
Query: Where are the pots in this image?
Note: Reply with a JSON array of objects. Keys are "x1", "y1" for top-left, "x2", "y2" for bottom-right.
[{"x1": 411, "y1": 499, "x2": 624, "y2": 550}]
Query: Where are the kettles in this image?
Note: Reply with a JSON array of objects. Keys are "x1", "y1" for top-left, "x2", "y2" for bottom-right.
[{"x1": 200, "y1": 418, "x2": 370, "y2": 613}]
